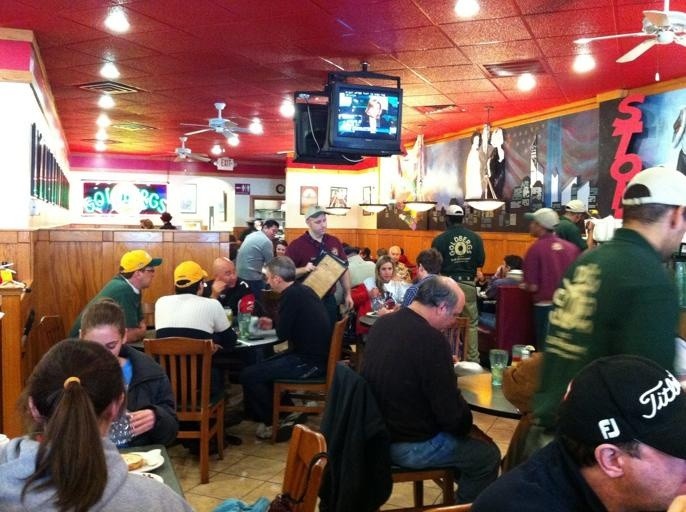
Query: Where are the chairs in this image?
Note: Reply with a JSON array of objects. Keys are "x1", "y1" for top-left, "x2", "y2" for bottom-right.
[
  {"x1": 266, "y1": 312, "x2": 350, "y2": 444},
  {"x1": 440, "y1": 314, "x2": 470, "y2": 361},
  {"x1": 477, "y1": 284, "x2": 529, "y2": 353},
  {"x1": 34, "y1": 314, "x2": 66, "y2": 350},
  {"x1": 140, "y1": 335, "x2": 229, "y2": 484},
  {"x1": 324, "y1": 364, "x2": 461, "y2": 508},
  {"x1": 269, "y1": 423, "x2": 328, "y2": 511}
]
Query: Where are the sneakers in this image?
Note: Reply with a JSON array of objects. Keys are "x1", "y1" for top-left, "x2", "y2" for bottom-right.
[
  {"x1": 276, "y1": 413, "x2": 307, "y2": 443},
  {"x1": 256, "y1": 425, "x2": 274, "y2": 439}
]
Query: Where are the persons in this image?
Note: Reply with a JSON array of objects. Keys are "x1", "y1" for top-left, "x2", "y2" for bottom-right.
[
  {"x1": 0, "y1": 339, "x2": 197, "y2": 512},
  {"x1": 354, "y1": 98, "x2": 392, "y2": 140},
  {"x1": 469, "y1": 355, "x2": 686, "y2": 512},
  {"x1": 388, "y1": 245, "x2": 412, "y2": 284},
  {"x1": 202, "y1": 257, "x2": 256, "y2": 314},
  {"x1": 553, "y1": 199, "x2": 595, "y2": 253},
  {"x1": 140, "y1": 218, "x2": 155, "y2": 229},
  {"x1": 347, "y1": 247, "x2": 375, "y2": 286},
  {"x1": 517, "y1": 208, "x2": 582, "y2": 353},
  {"x1": 375, "y1": 249, "x2": 464, "y2": 357},
  {"x1": 69, "y1": 249, "x2": 163, "y2": 345},
  {"x1": 377, "y1": 249, "x2": 387, "y2": 258},
  {"x1": 480, "y1": 128, "x2": 507, "y2": 200},
  {"x1": 500, "y1": 167, "x2": 686, "y2": 474},
  {"x1": 391, "y1": 148, "x2": 425, "y2": 202},
  {"x1": 274, "y1": 240, "x2": 288, "y2": 257},
  {"x1": 285, "y1": 205, "x2": 354, "y2": 321},
  {"x1": 159, "y1": 212, "x2": 176, "y2": 229},
  {"x1": 399, "y1": 248, "x2": 412, "y2": 264},
  {"x1": 465, "y1": 132, "x2": 482, "y2": 199},
  {"x1": 431, "y1": 205, "x2": 487, "y2": 364},
  {"x1": 239, "y1": 255, "x2": 332, "y2": 439},
  {"x1": 78, "y1": 297, "x2": 178, "y2": 448},
  {"x1": 342, "y1": 242, "x2": 349, "y2": 248},
  {"x1": 349, "y1": 275, "x2": 501, "y2": 505},
  {"x1": 237, "y1": 217, "x2": 258, "y2": 246},
  {"x1": 351, "y1": 255, "x2": 412, "y2": 346},
  {"x1": 361, "y1": 245, "x2": 377, "y2": 262},
  {"x1": 345, "y1": 246, "x2": 353, "y2": 259},
  {"x1": 235, "y1": 220, "x2": 279, "y2": 296},
  {"x1": 475, "y1": 255, "x2": 525, "y2": 332},
  {"x1": 154, "y1": 261, "x2": 238, "y2": 456}
]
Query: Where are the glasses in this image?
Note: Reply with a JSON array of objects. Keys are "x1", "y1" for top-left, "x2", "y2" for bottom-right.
[{"x1": 138, "y1": 268, "x2": 156, "y2": 275}]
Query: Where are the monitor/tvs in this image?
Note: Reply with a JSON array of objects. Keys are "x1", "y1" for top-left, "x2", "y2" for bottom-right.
[
  {"x1": 329, "y1": 84, "x2": 403, "y2": 153},
  {"x1": 291, "y1": 101, "x2": 361, "y2": 165}
]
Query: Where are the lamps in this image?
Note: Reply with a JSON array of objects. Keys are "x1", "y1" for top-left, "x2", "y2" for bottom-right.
[
  {"x1": 403, "y1": 122, "x2": 437, "y2": 213},
  {"x1": 325, "y1": 206, "x2": 352, "y2": 215},
  {"x1": 358, "y1": 204, "x2": 387, "y2": 213},
  {"x1": 464, "y1": 106, "x2": 506, "y2": 211}
]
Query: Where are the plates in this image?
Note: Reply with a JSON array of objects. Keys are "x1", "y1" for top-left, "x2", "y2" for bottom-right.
[
  {"x1": 366, "y1": 311, "x2": 380, "y2": 318},
  {"x1": 126, "y1": 451, "x2": 165, "y2": 473},
  {"x1": 128, "y1": 473, "x2": 164, "y2": 484}
]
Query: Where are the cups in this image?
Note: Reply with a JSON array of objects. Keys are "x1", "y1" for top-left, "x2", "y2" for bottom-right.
[
  {"x1": 513, "y1": 345, "x2": 530, "y2": 367},
  {"x1": 490, "y1": 349, "x2": 508, "y2": 386},
  {"x1": 238, "y1": 311, "x2": 252, "y2": 338}
]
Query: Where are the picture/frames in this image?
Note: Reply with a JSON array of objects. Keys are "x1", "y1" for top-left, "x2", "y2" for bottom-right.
[
  {"x1": 180, "y1": 182, "x2": 199, "y2": 214},
  {"x1": 328, "y1": 186, "x2": 349, "y2": 216},
  {"x1": 362, "y1": 185, "x2": 373, "y2": 216},
  {"x1": 300, "y1": 186, "x2": 319, "y2": 216}
]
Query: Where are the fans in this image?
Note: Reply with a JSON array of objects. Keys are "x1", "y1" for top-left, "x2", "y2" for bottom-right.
[
  {"x1": 180, "y1": 103, "x2": 250, "y2": 138},
  {"x1": 155, "y1": 138, "x2": 210, "y2": 164},
  {"x1": 573, "y1": 0, "x2": 686, "y2": 64}
]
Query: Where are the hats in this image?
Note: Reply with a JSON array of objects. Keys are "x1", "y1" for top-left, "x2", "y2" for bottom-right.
[
  {"x1": 621, "y1": 163, "x2": 686, "y2": 208},
  {"x1": 445, "y1": 204, "x2": 464, "y2": 216},
  {"x1": 564, "y1": 352, "x2": 686, "y2": 461},
  {"x1": 118, "y1": 248, "x2": 163, "y2": 276},
  {"x1": 524, "y1": 208, "x2": 561, "y2": 231},
  {"x1": 565, "y1": 199, "x2": 592, "y2": 219},
  {"x1": 304, "y1": 205, "x2": 331, "y2": 220},
  {"x1": 173, "y1": 259, "x2": 209, "y2": 288}
]
